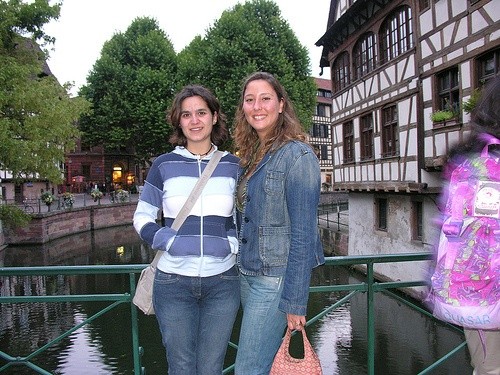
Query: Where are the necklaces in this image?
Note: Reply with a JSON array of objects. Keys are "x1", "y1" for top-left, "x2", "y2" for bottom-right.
[{"x1": 185, "y1": 144, "x2": 212, "y2": 156}]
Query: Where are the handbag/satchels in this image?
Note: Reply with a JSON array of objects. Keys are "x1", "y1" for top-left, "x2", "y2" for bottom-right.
[
  {"x1": 132, "y1": 267, "x2": 158, "y2": 315},
  {"x1": 270, "y1": 325, "x2": 321, "y2": 375}
]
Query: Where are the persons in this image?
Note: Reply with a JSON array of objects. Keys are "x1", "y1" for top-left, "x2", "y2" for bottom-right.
[
  {"x1": 230, "y1": 73, "x2": 325, "y2": 375},
  {"x1": 420, "y1": 76, "x2": 500, "y2": 375},
  {"x1": 133, "y1": 87, "x2": 241, "y2": 375}
]
